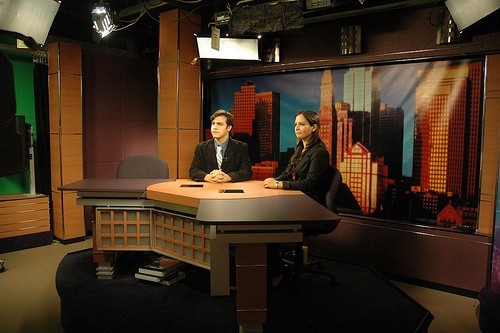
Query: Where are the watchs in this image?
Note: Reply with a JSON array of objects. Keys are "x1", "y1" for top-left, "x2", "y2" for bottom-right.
[{"x1": 275, "y1": 180, "x2": 279, "y2": 189}]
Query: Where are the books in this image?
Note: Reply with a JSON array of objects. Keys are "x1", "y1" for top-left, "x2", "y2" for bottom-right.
[{"x1": 135, "y1": 256, "x2": 187, "y2": 286}]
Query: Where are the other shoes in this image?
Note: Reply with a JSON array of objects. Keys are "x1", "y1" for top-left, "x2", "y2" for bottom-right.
[{"x1": 271, "y1": 261, "x2": 288, "y2": 292}]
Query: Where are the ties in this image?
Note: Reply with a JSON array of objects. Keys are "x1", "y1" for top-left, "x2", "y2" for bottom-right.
[{"x1": 215, "y1": 145, "x2": 223, "y2": 170}]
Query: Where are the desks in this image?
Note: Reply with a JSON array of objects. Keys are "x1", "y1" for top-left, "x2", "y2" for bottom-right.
[{"x1": 57, "y1": 179, "x2": 340, "y2": 333}]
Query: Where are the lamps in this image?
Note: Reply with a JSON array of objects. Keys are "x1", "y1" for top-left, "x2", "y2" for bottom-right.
[{"x1": 91, "y1": 0, "x2": 119, "y2": 38}]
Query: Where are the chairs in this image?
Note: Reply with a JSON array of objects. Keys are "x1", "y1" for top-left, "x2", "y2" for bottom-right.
[
  {"x1": 116, "y1": 155, "x2": 169, "y2": 178},
  {"x1": 302, "y1": 166, "x2": 342, "y2": 265}
]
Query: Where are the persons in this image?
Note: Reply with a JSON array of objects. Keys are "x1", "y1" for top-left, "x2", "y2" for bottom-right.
[
  {"x1": 264, "y1": 110, "x2": 330, "y2": 290},
  {"x1": 189, "y1": 109, "x2": 252, "y2": 184}
]
